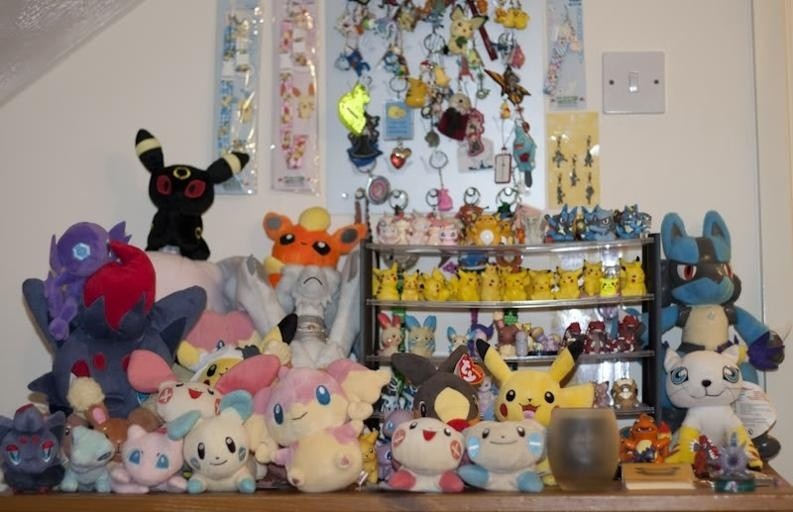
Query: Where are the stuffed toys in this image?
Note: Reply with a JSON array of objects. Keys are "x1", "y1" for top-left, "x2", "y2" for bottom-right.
[
  {"x1": 377, "y1": 337, "x2": 593, "y2": 494},
  {"x1": 134, "y1": 128, "x2": 249, "y2": 261},
  {"x1": 0, "y1": 205, "x2": 394, "y2": 495},
  {"x1": 659, "y1": 212, "x2": 785, "y2": 477},
  {"x1": 335, "y1": 0, "x2": 539, "y2": 204},
  {"x1": 0, "y1": 204, "x2": 783, "y2": 495}
]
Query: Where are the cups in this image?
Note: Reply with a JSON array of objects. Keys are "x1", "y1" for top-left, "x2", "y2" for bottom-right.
[{"x1": 547, "y1": 407, "x2": 619, "y2": 494}]
